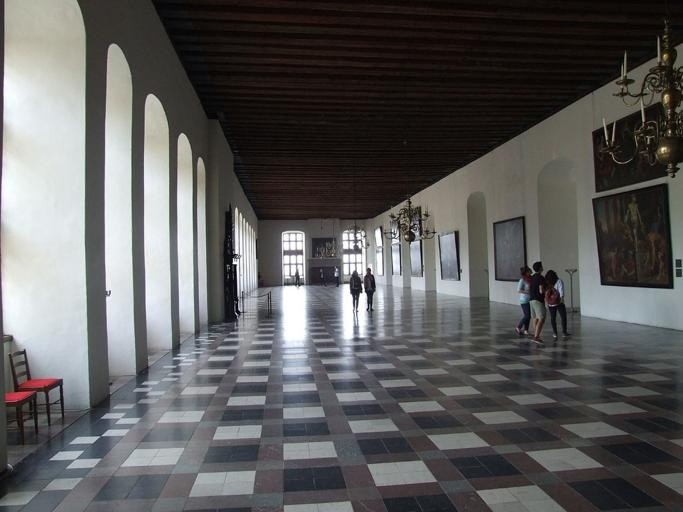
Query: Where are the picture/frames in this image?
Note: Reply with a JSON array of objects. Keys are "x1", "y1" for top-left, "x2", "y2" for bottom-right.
[
  {"x1": 390, "y1": 219, "x2": 402, "y2": 276},
  {"x1": 591, "y1": 102, "x2": 675, "y2": 194},
  {"x1": 408, "y1": 204, "x2": 423, "y2": 278},
  {"x1": 437, "y1": 230, "x2": 461, "y2": 282},
  {"x1": 591, "y1": 182, "x2": 674, "y2": 291},
  {"x1": 491, "y1": 215, "x2": 530, "y2": 283}
]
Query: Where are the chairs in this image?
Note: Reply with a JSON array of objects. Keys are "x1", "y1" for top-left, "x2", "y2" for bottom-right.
[
  {"x1": 7, "y1": 346, "x2": 66, "y2": 428},
  {"x1": 6, "y1": 371, "x2": 41, "y2": 444}
]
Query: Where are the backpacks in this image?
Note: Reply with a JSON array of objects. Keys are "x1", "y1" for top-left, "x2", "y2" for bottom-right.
[{"x1": 544, "y1": 280, "x2": 561, "y2": 305}]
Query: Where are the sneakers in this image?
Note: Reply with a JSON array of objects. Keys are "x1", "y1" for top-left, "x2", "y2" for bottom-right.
[
  {"x1": 514, "y1": 327, "x2": 531, "y2": 336},
  {"x1": 562, "y1": 332, "x2": 573, "y2": 338},
  {"x1": 552, "y1": 334, "x2": 558, "y2": 339},
  {"x1": 531, "y1": 334, "x2": 544, "y2": 346}
]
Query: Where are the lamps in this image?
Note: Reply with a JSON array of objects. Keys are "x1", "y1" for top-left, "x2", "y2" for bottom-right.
[
  {"x1": 343, "y1": 216, "x2": 370, "y2": 251},
  {"x1": 382, "y1": 186, "x2": 437, "y2": 244},
  {"x1": 598, "y1": 6, "x2": 681, "y2": 182}
]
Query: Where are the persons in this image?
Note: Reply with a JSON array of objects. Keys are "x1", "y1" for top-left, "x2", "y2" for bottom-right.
[
  {"x1": 624, "y1": 195, "x2": 643, "y2": 251},
  {"x1": 349, "y1": 271, "x2": 362, "y2": 313},
  {"x1": 514, "y1": 265, "x2": 532, "y2": 336},
  {"x1": 318, "y1": 268, "x2": 327, "y2": 287},
  {"x1": 294, "y1": 269, "x2": 300, "y2": 286},
  {"x1": 362, "y1": 268, "x2": 376, "y2": 313},
  {"x1": 543, "y1": 269, "x2": 572, "y2": 339},
  {"x1": 527, "y1": 262, "x2": 550, "y2": 346},
  {"x1": 333, "y1": 266, "x2": 340, "y2": 288}
]
